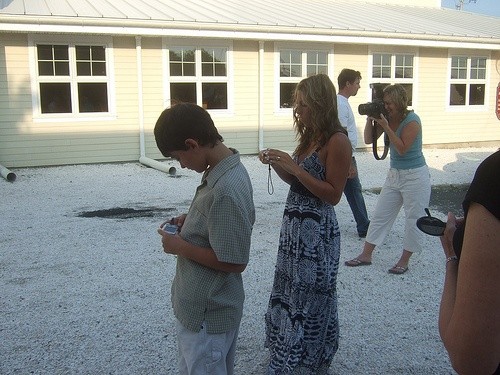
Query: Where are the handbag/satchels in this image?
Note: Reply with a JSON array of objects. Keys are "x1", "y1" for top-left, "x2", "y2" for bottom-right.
[{"x1": 347, "y1": 160, "x2": 356, "y2": 178}]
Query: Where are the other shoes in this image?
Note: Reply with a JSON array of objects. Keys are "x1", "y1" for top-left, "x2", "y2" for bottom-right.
[{"x1": 358, "y1": 231, "x2": 367, "y2": 238}]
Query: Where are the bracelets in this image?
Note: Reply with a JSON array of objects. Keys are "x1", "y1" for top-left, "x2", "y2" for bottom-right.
[{"x1": 446, "y1": 255, "x2": 457, "y2": 264}]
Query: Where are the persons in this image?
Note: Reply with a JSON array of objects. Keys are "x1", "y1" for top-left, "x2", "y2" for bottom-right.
[
  {"x1": 258, "y1": 74, "x2": 353, "y2": 375},
  {"x1": 344, "y1": 84, "x2": 431, "y2": 274},
  {"x1": 440, "y1": 148, "x2": 500, "y2": 375},
  {"x1": 336, "y1": 69, "x2": 371, "y2": 237},
  {"x1": 153, "y1": 102, "x2": 256, "y2": 375}
]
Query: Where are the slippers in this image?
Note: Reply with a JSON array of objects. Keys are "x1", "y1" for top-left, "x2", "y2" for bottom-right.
[
  {"x1": 388, "y1": 264, "x2": 408, "y2": 273},
  {"x1": 345, "y1": 257, "x2": 371, "y2": 266}
]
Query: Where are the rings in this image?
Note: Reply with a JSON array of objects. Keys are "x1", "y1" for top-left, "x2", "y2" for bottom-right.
[{"x1": 276, "y1": 156, "x2": 281, "y2": 160}]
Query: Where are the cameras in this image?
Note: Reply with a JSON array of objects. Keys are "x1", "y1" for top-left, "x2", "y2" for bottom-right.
[
  {"x1": 161, "y1": 222, "x2": 179, "y2": 235},
  {"x1": 260, "y1": 148, "x2": 276, "y2": 164},
  {"x1": 358, "y1": 98, "x2": 387, "y2": 119}
]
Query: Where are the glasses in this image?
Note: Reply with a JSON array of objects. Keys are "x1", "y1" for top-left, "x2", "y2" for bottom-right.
[{"x1": 416, "y1": 208, "x2": 465, "y2": 236}]
